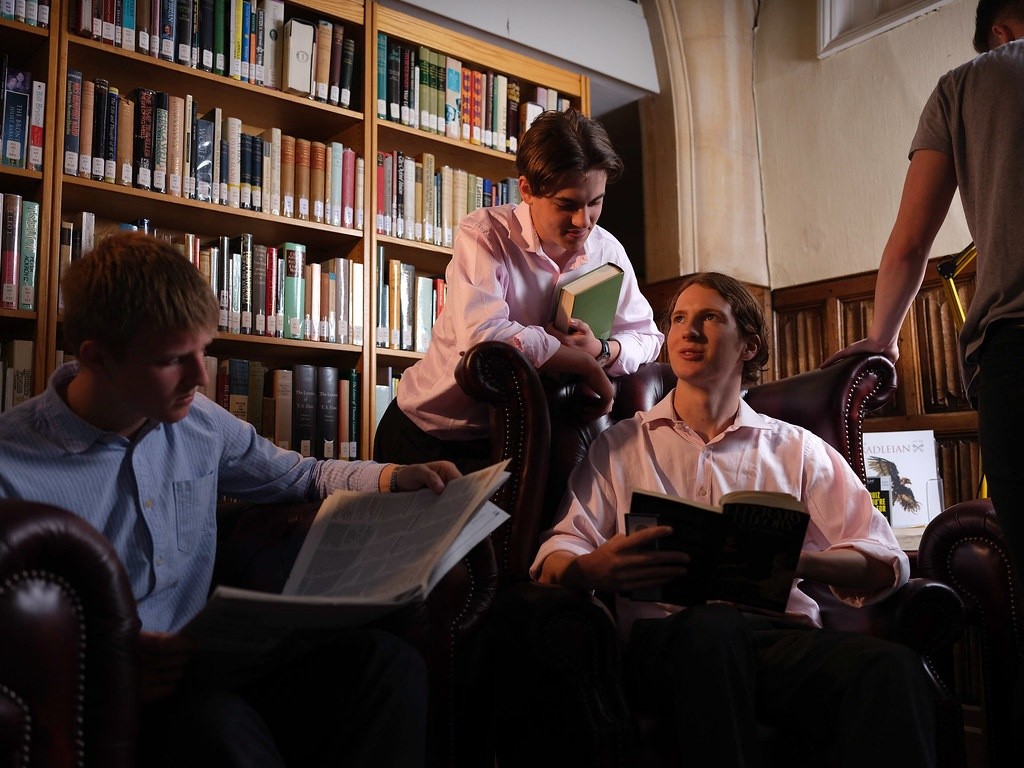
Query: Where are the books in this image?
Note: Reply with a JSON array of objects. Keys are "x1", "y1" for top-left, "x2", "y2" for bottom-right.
[
  {"x1": 628, "y1": 490, "x2": 812, "y2": 615},
  {"x1": 0, "y1": 0, "x2": 581, "y2": 463},
  {"x1": 557, "y1": 262, "x2": 624, "y2": 344}
]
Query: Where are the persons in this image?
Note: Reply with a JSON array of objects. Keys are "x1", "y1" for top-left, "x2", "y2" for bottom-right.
[
  {"x1": 528, "y1": 272, "x2": 937, "y2": 768},
  {"x1": 819, "y1": 0, "x2": 1024, "y2": 768},
  {"x1": 373, "y1": 106, "x2": 665, "y2": 480},
  {"x1": 0, "y1": 229, "x2": 461, "y2": 655}
]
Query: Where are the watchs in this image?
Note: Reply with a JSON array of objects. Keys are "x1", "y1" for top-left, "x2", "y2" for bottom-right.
[{"x1": 593, "y1": 336, "x2": 612, "y2": 371}]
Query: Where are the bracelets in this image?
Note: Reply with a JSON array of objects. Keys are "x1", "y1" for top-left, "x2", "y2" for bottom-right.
[{"x1": 389, "y1": 463, "x2": 408, "y2": 494}]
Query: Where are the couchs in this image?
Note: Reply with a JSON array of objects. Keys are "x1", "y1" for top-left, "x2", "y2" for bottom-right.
[
  {"x1": 454, "y1": 340, "x2": 965, "y2": 768},
  {"x1": 917, "y1": 498, "x2": 1024, "y2": 768},
  {"x1": 1, "y1": 501, "x2": 499, "y2": 768}
]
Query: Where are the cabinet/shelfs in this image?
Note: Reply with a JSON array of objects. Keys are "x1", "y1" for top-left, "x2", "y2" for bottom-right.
[
  {"x1": 369, "y1": 3, "x2": 591, "y2": 461},
  {"x1": 1, "y1": 1, "x2": 59, "y2": 412},
  {"x1": 45, "y1": 1, "x2": 372, "y2": 461}
]
